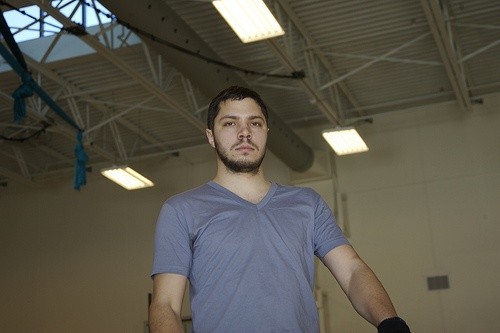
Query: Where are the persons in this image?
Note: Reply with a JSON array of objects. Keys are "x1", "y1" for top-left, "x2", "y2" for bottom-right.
[{"x1": 149, "y1": 86, "x2": 411, "y2": 333}]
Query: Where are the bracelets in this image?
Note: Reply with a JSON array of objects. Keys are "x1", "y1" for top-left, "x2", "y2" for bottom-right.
[{"x1": 377, "y1": 316, "x2": 410, "y2": 333}]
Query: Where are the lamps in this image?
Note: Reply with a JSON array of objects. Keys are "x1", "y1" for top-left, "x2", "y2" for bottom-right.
[
  {"x1": 320, "y1": 124, "x2": 370, "y2": 157},
  {"x1": 99, "y1": 90, "x2": 156, "y2": 192},
  {"x1": 211, "y1": 0, "x2": 286, "y2": 45}
]
